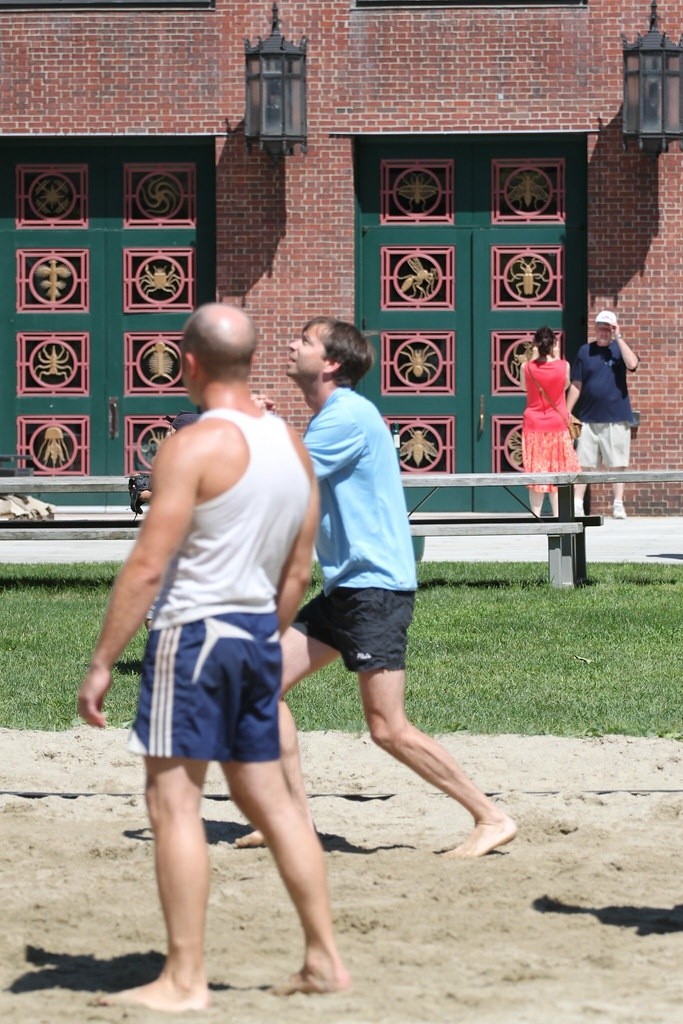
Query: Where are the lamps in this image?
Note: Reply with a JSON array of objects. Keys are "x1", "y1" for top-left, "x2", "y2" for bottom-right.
[
  {"x1": 619, "y1": 0, "x2": 683, "y2": 154},
  {"x1": 242, "y1": 1, "x2": 310, "y2": 159}
]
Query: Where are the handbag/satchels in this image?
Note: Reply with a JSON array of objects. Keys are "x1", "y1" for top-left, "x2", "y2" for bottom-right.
[{"x1": 569, "y1": 424, "x2": 580, "y2": 440}]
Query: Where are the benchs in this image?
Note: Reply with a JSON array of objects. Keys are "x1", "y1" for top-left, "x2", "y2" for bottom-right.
[
  {"x1": 408, "y1": 515, "x2": 605, "y2": 589},
  {"x1": 0, "y1": 518, "x2": 140, "y2": 539}
]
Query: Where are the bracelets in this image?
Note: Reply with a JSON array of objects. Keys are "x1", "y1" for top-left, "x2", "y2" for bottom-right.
[{"x1": 615, "y1": 336, "x2": 621, "y2": 341}]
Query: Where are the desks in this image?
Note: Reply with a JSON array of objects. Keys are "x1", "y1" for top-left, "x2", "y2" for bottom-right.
[
  {"x1": 0, "y1": 477, "x2": 135, "y2": 492},
  {"x1": 401, "y1": 469, "x2": 683, "y2": 587}
]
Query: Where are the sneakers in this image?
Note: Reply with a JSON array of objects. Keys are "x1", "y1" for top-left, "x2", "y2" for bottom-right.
[{"x1": 613, "y1": 499, "x2": 626, "y2": 518}]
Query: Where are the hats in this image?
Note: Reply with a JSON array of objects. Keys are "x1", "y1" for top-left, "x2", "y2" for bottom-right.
[
  {"x1": 172, "y1": 413, "x2": 200, "y2": 431},
  {"x1": 595, "y1": 310, "x2": 617, "y2": 326}
]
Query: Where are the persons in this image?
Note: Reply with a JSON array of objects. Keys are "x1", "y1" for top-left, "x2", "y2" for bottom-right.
[
  {"x1": 566, "y1": 311, "x2": 641, "y2": 519},
  {"x1": 520, "y1": 326, "x2": 582, "y2": 517},
  {"x1": 78, "y1": 304, "x2": 348, "y2": 1013},
  {"x1": 127, "y1": 315, "x2": 518, "y2": 860}
]
